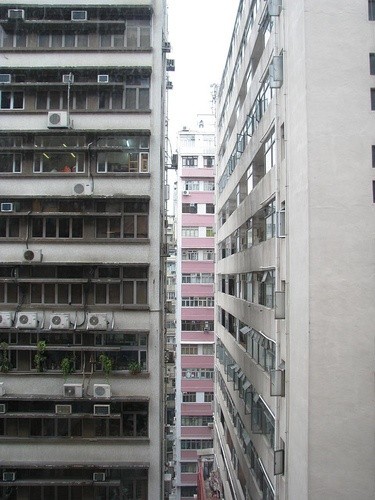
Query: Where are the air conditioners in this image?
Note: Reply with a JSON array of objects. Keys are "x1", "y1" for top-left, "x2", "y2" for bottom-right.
[
  {"x1": 97, "y1": 74, "x2": 109, "y2": 83},
  {"x1": 0, "y1": 312, "x2": 108, "y2": 330},
  {"x1": 62, "y1": 74, "x2": 74, "y2": 83},
  {"x1": 93, "y1": 383, "x2": 111, "y2": 398},
  {"x1": 7, "y1": 9, "x2": 87, "y2": 21},
  {"x1": 72, "y1": 182, "x2": 92, "y2": 195},
  {"x1": 0, "y1": 402, "x2": 110, "y2": 482},
  {"x1": 183, "y1": 190, "x2": 190, "y2": 196},
  {"x1": 62, "y1": 384, "x2": 83, "y2": 398},
  {"x1": 21, "y1": 249, "x2": 42, "y2": 263},
  {"x1": 0, "y1": 74, "x2": 11, "y2": 83},
  {"x1": 1, "y1": 203, "x2": 16, "y2": 212},
  {"x1": 47, "y1": 111, "x2": 67, "y2": 127},
  {"x1": 163, "y1": 41, "x2": 175, "y2": 90}
]
